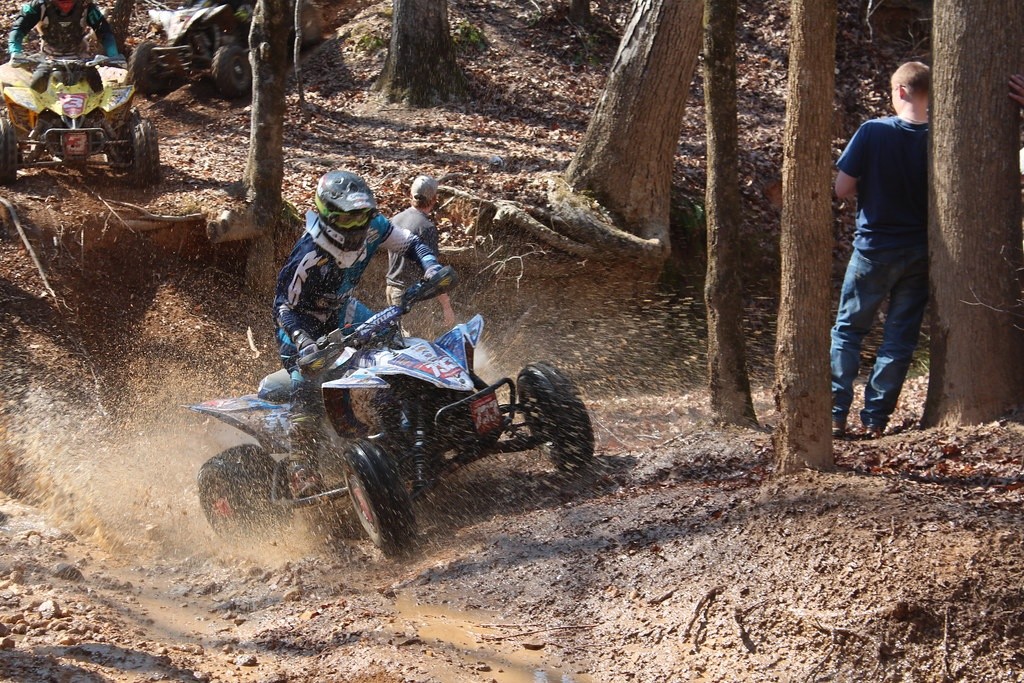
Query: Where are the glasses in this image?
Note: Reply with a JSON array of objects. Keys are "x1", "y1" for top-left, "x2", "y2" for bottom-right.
[{"x1": 315, "y1": 191, "x2": 372, "y2": 228}]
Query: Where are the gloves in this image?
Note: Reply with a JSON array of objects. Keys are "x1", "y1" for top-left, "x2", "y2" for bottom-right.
[
  {"x1": 423, "y1": 260, "x2": 442, "y2": 282},
  {"x1": 296, "y1": 333, "x2": 319, "y2": 359}
]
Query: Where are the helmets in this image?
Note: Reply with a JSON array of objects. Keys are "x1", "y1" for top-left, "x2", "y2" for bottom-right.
[{"x1": 316, "y1": 171, "x2": 376, "y2": 252}]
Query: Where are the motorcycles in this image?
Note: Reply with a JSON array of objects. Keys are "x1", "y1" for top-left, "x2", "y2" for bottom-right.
[
  {"x1": 130, "y1": 0, "x2": 326, "y2": 100},
  {"x1": 177, "y1": 265, "x2": 595, "y2": 558},
  {"x1": 0, "y1": 50, "x2": 161, "y2": 191}
]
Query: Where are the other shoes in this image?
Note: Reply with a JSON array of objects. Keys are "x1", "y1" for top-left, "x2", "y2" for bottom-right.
[
  {"x1": 832, "y1": 421, "x2": 845, "y2": 436},
  {"x1": 865, "y1": 426, "x2": 881, "y2": 437}
]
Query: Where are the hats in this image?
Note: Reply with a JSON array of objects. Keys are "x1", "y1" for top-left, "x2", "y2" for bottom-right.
[{"x1": 411, "y1": 175, "x2": 437, "y2": 201}]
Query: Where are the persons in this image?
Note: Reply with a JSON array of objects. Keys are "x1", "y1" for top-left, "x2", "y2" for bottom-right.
[
  {"x1": 831, "y1": 61, "x2": 928, "y2": 433},
  {"x1": 1008, "y1": 74, "x2": 1024, "y2": 105},
  {"x1": 271, "y1": 169, "x2": 454, "y2": 499},
  {"x1": 8, "y1": 0, "x2": 127, "y2": 94}
]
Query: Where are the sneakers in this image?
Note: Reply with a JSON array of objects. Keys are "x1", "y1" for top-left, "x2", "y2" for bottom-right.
[{"x1": 286, "y1": 466, "x2": 321, "y2": 499}]
